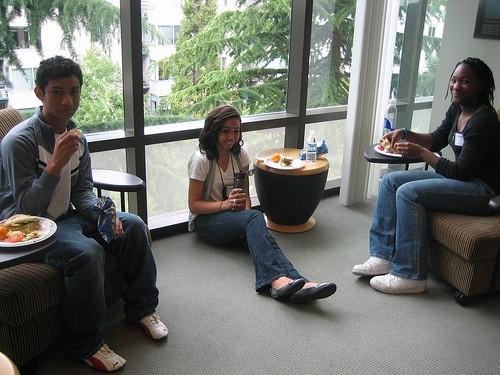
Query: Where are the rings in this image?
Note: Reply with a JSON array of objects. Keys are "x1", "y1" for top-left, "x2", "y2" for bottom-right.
[
  {"x1": 234, "y1": 199, "x2": 236, "y2": 203},
  {"x1": 402, "y1": 152, "x2": 403, "y2": 155}
]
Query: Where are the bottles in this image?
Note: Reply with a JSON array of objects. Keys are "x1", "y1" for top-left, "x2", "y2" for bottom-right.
[
  {"x1": 305, "y1": 130, "x2": 317, "y2": 166},
  {"x1": 234, "y1": 169, "x2": 248, "y2": 211},
  {"x1": 382, "y1": 98, "x2": 397, "y2": 135}
]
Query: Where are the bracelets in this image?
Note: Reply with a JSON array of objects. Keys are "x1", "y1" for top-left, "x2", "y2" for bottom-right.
[
  {"x1": 402, "y1": 128, "x2": 407, "y2": 140},
  {"x1": 220, "y1": 201, "x2": 223, "y2": 211}
]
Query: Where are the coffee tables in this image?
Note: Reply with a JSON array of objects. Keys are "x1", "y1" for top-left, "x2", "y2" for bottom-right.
[
  {"x1": 1, "y1": 236, "x2": 57, "y2": 270},
  {"x1": 91, "y1": 168, "x2": 145, "y2": 212},
  {"x1": 364, "y1": 142, "x2": 443, "y2": 171},
  {"x1": 253, "y1": 148, "x2": 330, "y2": 234}
]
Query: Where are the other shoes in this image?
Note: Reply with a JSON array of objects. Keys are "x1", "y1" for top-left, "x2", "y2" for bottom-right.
[
  {"x1": 290, "y1": 281, "x2": 336, "y2": 305},
  {"x1": 269, "y1": 278, "x2": 306, "y2": 300}
]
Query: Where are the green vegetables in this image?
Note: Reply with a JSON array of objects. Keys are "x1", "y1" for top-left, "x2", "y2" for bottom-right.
[{"x1": 280, "y1": 157, "x2": 290, "y2": 167}]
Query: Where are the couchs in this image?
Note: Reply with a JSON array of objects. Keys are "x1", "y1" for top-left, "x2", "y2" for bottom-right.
[
  {"x1": 0, "y1": 107, "x2": 153, "y2": 369},
  {"x1": 426, "y1": 107, "x2": 500, "y2": 307}
]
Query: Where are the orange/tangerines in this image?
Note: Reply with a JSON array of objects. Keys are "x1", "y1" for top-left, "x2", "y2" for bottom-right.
[
  {"x1": 0, "y1": 226, "x2": 9, "y2": 240},
  {"x1": 272, "y1": 153, "x2": 280, "y2": 162}
]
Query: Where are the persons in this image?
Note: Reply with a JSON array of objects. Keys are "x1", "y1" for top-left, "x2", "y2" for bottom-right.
[
  {"x1": 187, "y1": 106, "x2": 337, "y2": 304},
  {"x1": 352, "y1": 57, "x2": 500, "y2": 294},
  {"x1": 0, "y1": 56, "x2": 168, "y2": 371}
]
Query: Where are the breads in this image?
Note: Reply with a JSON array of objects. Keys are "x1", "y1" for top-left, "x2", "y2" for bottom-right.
[{"x1": 380, "y1": 138, "x2": 398, "y2": 154}]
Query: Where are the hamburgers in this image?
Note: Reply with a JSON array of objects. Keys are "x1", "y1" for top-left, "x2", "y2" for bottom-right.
[{"x1": 3, "y1": 214, "x2": 40, "y2": 231}]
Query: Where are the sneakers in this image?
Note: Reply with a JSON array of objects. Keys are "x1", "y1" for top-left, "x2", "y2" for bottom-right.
[
  {"x1": 352, "y1": 256, "x2": 393, "y2": 276},
  {"x1": 80, "y1": 341, "x2": 127, "y2": 372},
  {"x1": 139, "y1": 313, "x2": 169, "y2": 341},
  {"x1": 369, "y1": 272, "x2": 428, "y2": 294}
]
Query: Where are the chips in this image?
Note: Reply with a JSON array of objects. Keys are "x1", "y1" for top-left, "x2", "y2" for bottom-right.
[{"x1": 70, "y1": 128, "x2": 84, "y2": 138}]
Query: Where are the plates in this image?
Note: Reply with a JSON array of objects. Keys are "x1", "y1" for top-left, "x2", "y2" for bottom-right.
[
  {"x1": 373, "y1": 145, "x2": 402, "y2": 158},
  {"x1": 0, "y1": 217, "x2": 58, "y2": 247},
  {"x1": 263, "y1": 156, "x2": 305, "y2": 170}
]
